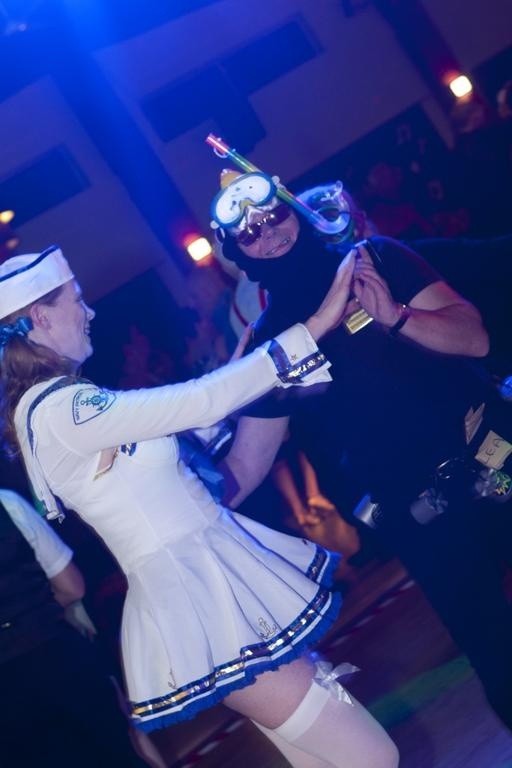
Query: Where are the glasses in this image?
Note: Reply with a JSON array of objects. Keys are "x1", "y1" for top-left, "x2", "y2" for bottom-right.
[{"x1": 235, "y1": 203, "x2": 289, "y2": 246}]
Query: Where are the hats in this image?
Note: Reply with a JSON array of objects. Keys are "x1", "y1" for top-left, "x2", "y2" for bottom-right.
[{"x1": 0, "y1": 245, "x2": 74, "y2": 318}]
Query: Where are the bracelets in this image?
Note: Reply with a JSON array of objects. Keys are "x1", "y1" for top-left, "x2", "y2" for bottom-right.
[{"x1": 389, "y1": 302, "x2": 409, "y2": 337}]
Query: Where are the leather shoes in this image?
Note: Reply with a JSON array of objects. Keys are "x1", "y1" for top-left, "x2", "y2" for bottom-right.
[{"x1": 298, "y1": 495, "x2": 336, "y2": 526}]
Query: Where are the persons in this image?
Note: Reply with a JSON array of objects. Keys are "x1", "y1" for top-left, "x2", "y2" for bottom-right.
[
  {"x1": 275, "y1": 448, "x2": 335, "y2": 527},
  {"x1": 0, "y1": 486, "x2": 162, "y2": 767},
  {"x1": 203, "y1": 171, "x2": 512, "y2": 733},
  {"x1": 1, "y1": 241, "x2": 407, "y2": 767}
]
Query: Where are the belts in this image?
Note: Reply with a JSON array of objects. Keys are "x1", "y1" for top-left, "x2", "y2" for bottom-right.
[{"x1": 432, "y1": 417, "x2": 491, "y2": 488}]
